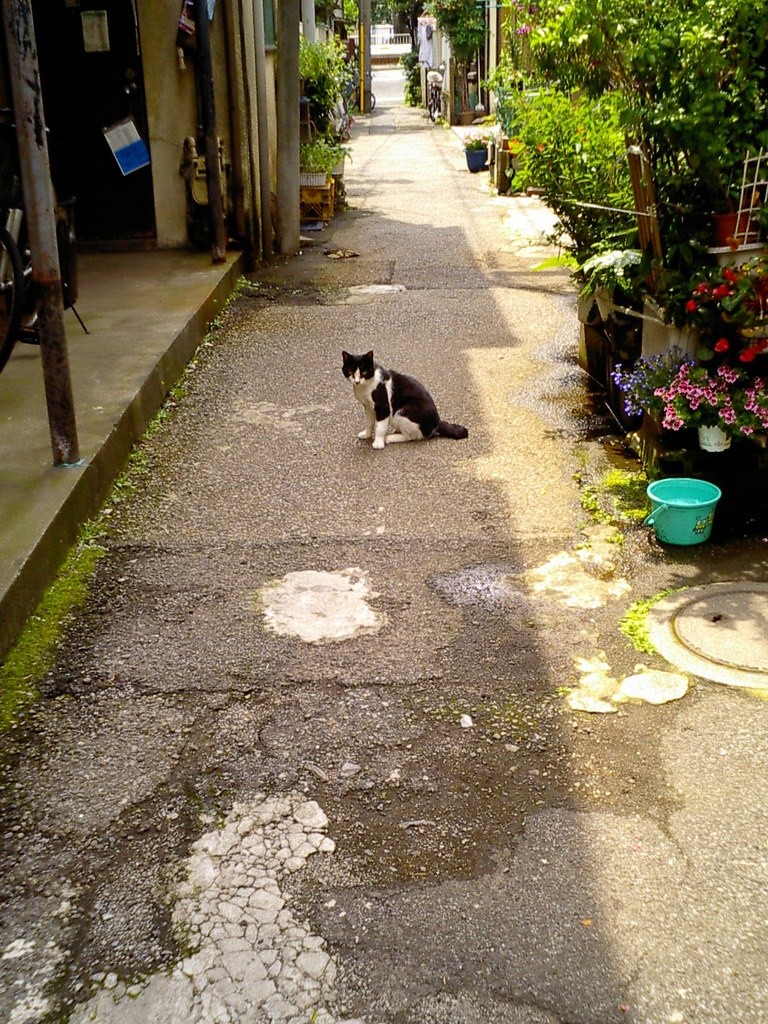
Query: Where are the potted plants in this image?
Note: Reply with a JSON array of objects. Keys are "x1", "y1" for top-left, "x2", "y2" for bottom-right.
[
  {"x1": 300, "y1": 124, "x2": 352, "y2": 186},
  {"x1": 492, "y1": 89, "x2": 637, "y2": 327}
]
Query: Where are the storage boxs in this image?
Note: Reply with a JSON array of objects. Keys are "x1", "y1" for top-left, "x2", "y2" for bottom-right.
[{"x1": 301, "y1": 178, "x2": 335, "y2": 222}]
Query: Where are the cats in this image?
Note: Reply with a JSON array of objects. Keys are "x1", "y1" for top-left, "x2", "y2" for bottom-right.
[{"x1": 342, "y1": 351, "x2": 469, "y2": 450}]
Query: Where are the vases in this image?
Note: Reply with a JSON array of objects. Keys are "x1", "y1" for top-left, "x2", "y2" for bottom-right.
[
  {"x1": 458, "y1": 111, "x2": 474, "y2": 126},
  {"x1": 698, "y1": 423, "x2": 730, "y2": 451},
  {"x1": 707, "y1": 208, "x2": 768, "y2": 274},
  {"x1": 638, "y1": 288, "x2": 702, "y2": 368},
  {"x1": 464, "y1": 148, "x2": 488, "y2": 173}
]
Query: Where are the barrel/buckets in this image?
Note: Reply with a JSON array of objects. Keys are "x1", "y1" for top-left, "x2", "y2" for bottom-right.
[{"x1": 645, "y1": 478, "x2": 722, "y2": 545}]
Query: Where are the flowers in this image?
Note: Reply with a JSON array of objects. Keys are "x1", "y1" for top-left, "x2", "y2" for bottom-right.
[
  {"x1": 461, "y1": 131, "x2": 487, "y2": 150},
  {"x1": 611, "y1": 258, "x2": 768, "y2": 448}
]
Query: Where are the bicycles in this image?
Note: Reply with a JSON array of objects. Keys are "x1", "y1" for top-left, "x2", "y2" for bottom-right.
[
  {"x1": 420, "y1": 59, "x2": 447, "y2": 123},
  {"x1": 0, "y1": 106, "x2": 90, "y2": 375},
  {"x1": 346, "y1": 71, "x2": 376, "y2": 113}
]
